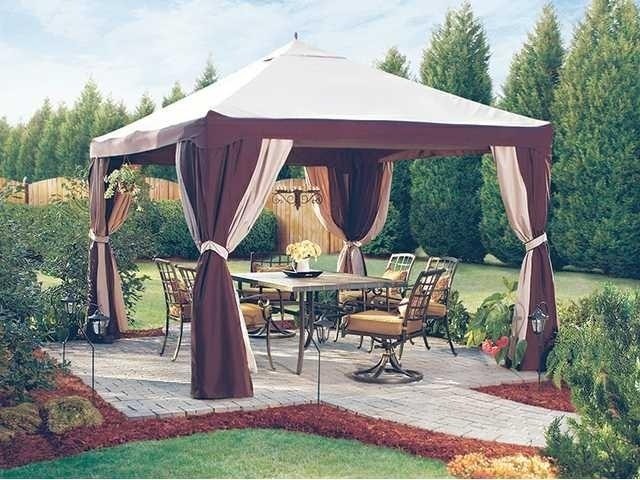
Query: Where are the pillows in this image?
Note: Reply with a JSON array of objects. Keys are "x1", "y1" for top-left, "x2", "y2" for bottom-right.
[
  {"x1": 433, "y1": 276, "x2": 449, "y2": 304},
  {"x1": 382, "y1": 268, "x2": 407, "y2": 297}
]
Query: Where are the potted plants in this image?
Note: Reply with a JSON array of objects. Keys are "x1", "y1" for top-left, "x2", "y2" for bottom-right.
[{"x1": 104, "y1": 163, "x2": 152, "y2": 212}]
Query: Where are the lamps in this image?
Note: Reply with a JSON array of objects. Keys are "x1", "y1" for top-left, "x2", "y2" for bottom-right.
[
  {"x1": 528, "y1": 304, "x2": 550, "y2": 335},
  {"x1": 272, "y1": 167, "x2": 323, "y2": 212},
  {"x1": 60, "y1": 291, "x2": 78, "y2": 317},
  {"x1": 88, "y1": 307, "x2": 110, "y2": 336}
]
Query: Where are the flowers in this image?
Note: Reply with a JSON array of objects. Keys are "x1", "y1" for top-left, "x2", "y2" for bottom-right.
[{"x1": 286, "y1": 240, "x2": 321, "y2": 263}]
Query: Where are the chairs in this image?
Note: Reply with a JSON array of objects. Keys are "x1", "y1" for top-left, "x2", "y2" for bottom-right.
[
  {"x1": 249, "y1": 252, "x2": 298, "y2": 328},
  {"x1": 174, "y1": 264, "x2": 277, "y2": 372},
  {"x1": 337, "y1": 253, "x2": 416, "y2": 310},
  {"x1": 339, "y1": 268, "x2": 445, "y2": 383},
  {"x1": 397, "y1": 257, "x2": 460, "y2": 357},
  {"x1": 401, "y1": 255, "x2": 440, "y2": 304},
  {"x1": 152, "y1": 258, "x2": 192, "y2": 362}
]
